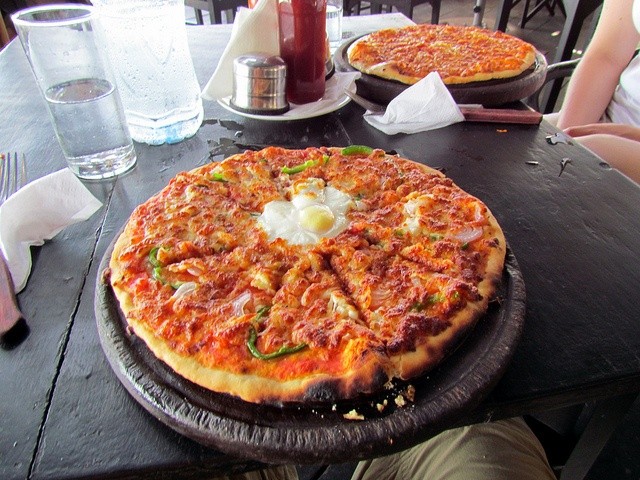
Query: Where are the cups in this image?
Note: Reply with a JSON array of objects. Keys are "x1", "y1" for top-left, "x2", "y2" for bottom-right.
[
  {"x1": 10, "y1": 5, "x2": 137, "y2": 182},
  {"x1": 325, "y1": 1, "x2": 343, "y2": 50}
]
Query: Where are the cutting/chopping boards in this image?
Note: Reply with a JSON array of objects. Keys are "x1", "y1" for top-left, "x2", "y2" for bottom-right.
[
  {"x1": 94, "y1": 145, "x2": 527, "y2": 463},
  {"x1": 333, "y1": 23, "x2": 548, "y2": 107}
]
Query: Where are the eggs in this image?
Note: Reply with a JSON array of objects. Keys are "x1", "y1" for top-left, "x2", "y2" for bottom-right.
[{"x1": 254, "y1": 185, "x2": 355, "y2": 249}]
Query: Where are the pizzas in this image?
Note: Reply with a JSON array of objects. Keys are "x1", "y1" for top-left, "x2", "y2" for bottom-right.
[
  {"x1": 108, "y1": 143, "x2": 507, "y2": 408},
  {"x1": 347, "y1": 23, "x2": 537, "y2": 85}
]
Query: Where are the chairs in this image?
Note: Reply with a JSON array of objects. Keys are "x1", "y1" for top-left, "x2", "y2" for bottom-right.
[{"x1": 527, "y1": 51, "x2": 635, "y2": 114}]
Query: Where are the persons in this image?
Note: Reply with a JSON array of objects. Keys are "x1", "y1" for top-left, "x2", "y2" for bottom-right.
[
  {"x1": 542, "y1": 0, "x2": 640, "y2": 188},
  {"x1": 349, "y1": 415, "x2": 558, "y2": 480}
]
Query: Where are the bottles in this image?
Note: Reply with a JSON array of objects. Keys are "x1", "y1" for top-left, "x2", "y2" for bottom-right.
[
  {"x1": 231, "y1": 53, "x2": 290, "y2": 114},
  {"x1": 278, "y1": 0, "x2": 325, "y2": 105},
  {"x1": 89, "y1": 0, "x2": 203, "y2": 147}
]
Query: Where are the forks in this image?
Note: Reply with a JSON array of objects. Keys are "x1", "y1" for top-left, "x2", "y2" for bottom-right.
[{"x1": 1, "y1": 152, "x2": 27, "y2": 203}]
